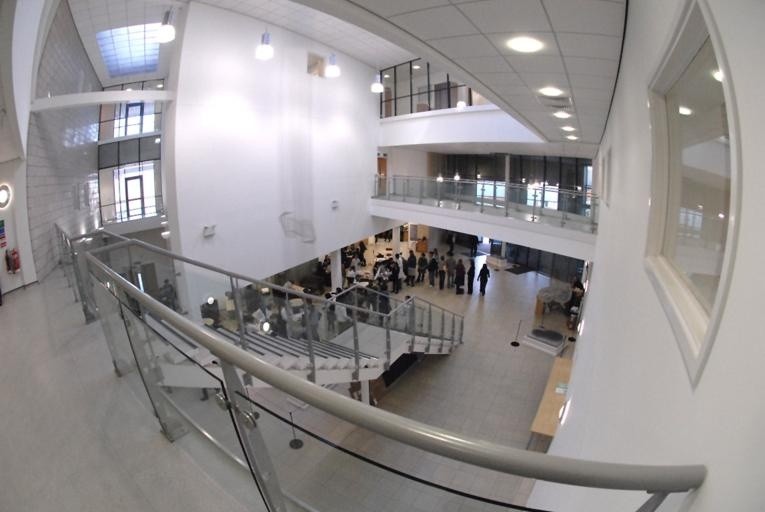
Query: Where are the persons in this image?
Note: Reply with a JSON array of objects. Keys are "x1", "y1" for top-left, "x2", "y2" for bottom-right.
[
  {"x1": 200, "y1": 225, "x2": 457, "y2": 342},
  {"x1": 566, "y1": 276, "x2": 584, "y2": 316},
  {"x1": 467, "y1": 259, "x2": 475, "y2": 294},
  {"x1": 478, "y1": 264, "x2": 490, "y2": 296},
  {"x1": 122, "y1": 279, "x2": 176, "y2": 315},
  {"x1": 470, "y1": 235, "x2": 479, "y2": 257},
  {"x1": 455, "y1": 259, "x2": 465, "y2": 294}
]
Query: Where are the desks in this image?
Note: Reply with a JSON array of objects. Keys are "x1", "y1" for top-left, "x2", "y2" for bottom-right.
[{"x1": 525, "y1": 357, "x2": 572, "y2": 450}]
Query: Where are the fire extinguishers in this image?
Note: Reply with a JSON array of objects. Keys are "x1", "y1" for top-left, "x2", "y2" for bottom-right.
[{"x1": 11, "y1": 247, "x2": 20, "y2": 269}]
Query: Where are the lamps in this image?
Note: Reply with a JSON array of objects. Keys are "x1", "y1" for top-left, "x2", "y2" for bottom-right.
[
  {"x1": 325, "y1": 54, "x2": 339, "y2": 78},
  {"x1": 255, "y1": 32, "x2": 272, "y2": 60},
  {"x1": 370, "y1": 71, "x2": 383, "y2": 93},
  {"x1": 157, "y1": 6, "x2": 175, "y2": 43}
]
